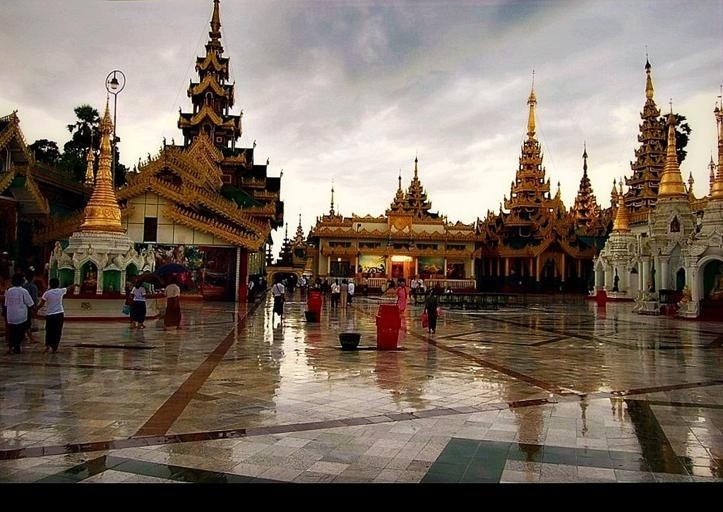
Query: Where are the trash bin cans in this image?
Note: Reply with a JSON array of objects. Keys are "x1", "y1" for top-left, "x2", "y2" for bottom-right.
[
  {"x1": 375, "y1": 304, "x2": 401, "y2": 350},
  {"x1": 596, "y1": 289, "x2": 606, "y2": 307},
  {"x1": 307, "y1": 291, "x2": 322, "y2": 322}
]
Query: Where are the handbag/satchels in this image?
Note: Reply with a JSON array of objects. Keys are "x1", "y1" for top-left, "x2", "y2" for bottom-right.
[
  {"x1": 122, "y1": 306, "x2": 130, "y2": 314},
  {"x1": 334, "y1": 287, "x2": 340, "y2": 293},
  {"x1": 421, "y1": 313, "x2": 428, "y2": 328},
  {"x1": 125, "y1": 293, "x2": 133, "y2": 305},
  {"x1": 436, "y1": 306, "x2": 440, "y2": 317}
]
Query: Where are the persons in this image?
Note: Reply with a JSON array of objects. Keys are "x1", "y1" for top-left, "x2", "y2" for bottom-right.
[
  {"x1": 269, "y1": 270, "x2": 454, "y2": 335},
  {"x1": 23, "y1": 271, "x2": 39, "y2": 344},
  {"x1": 129, "y1": 281, "x2": 146, "y2": 329},
  {"x1": 3, "y1": 273, "x2": 37, "y2": 355},
  {"x1": 125, "y1": 280, "x2": 136, "y2": 328},
  {"x1": 163, "y1": 276, "x2": 182, "y2": 331},
  {"x1": 35, "y1": 278, "x2": 80, "y2": 354}
]
